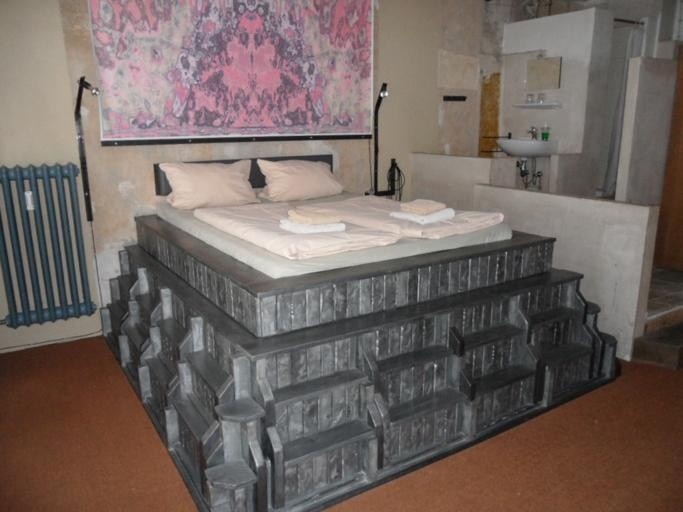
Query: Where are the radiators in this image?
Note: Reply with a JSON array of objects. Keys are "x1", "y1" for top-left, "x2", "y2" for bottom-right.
[{"x1": 0, "y1": 161, "x2": 97, "y2": 330}]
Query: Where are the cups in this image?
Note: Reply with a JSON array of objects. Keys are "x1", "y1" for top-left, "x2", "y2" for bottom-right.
[
  {"x1": 526, "y1": 93, "x2": 536, "y2": 104},
  {"x1": 536, "y1": 93, "x2": 546, "y2": 104}
]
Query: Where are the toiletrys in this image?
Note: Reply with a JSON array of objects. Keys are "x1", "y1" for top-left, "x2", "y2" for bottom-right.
[{"x1": 541, "y1": 122, "x2": 549, "y2": 141}]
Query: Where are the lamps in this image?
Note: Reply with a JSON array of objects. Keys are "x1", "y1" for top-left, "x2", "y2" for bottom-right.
[
  {"x1": 73, "y1": 75, "x2": 101, "y2": 223},
  {"x1": 364, "y1": 81, "x2": 396, "y2": 196}
]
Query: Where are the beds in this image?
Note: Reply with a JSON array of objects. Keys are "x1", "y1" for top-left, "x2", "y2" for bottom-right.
[{"x1": 104, "y1": 152, "x2": 618, "y2": 512}]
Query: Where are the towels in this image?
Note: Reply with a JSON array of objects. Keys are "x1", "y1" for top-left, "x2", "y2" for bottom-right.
[
  {"x1": 279, "y1": 204, "x2": 347, "y2": 235},
  {"x1": 388, "y1": 199, "x2": 456, "y2": 226}
]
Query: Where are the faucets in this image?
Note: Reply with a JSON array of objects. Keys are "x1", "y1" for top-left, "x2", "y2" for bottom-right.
[{"x1": 526, "y1": 126, "x2": 537, "y2": 140}]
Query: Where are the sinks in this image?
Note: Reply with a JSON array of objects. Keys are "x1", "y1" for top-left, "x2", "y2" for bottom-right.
[{"x1": 496, "y1": 138, "x2": 552, "y2": 156}]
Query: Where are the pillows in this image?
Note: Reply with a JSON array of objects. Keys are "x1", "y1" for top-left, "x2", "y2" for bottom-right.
[
  {"x1": 158, "y1": 158, "x2": 262, "y2": 212},
  {"x1": 255, "y1": 158, "x2": 345, "y2": 202}
]
